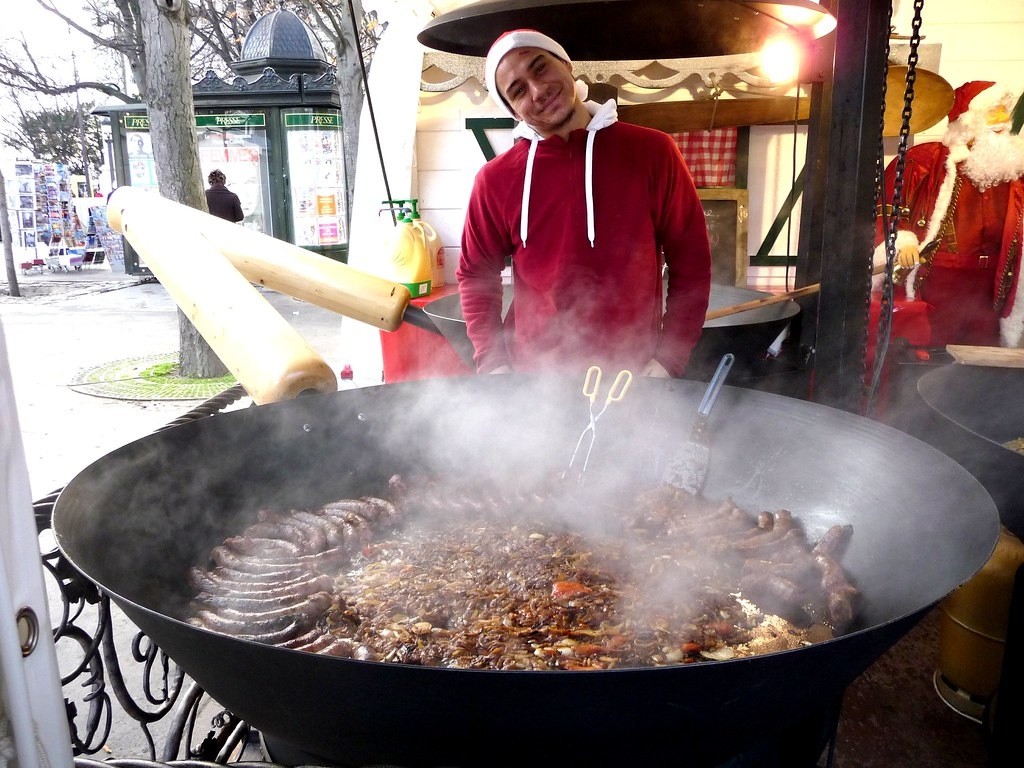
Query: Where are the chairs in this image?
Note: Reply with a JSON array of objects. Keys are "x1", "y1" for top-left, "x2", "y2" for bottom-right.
[
  {"x1": 92, "y1": 250, "x2": 108, "y2": 270},
  {"x1": 82, "y1": 252, "x2": 95, "y2": 271}
]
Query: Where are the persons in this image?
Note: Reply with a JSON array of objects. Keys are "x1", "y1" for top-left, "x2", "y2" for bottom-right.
[
  {"x1": 204, "y1": 168, "x2": 243, "y2": 222},
  {"x1": 810, "y1": 78, "x2": 1024, "y2": 423},
  {"x1": 456, "y1": 29, "x2": 711, "y2": 380}
]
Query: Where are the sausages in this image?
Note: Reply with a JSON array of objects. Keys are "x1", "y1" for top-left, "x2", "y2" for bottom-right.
[{"x1": 179, "y1": 473, "x2": 858, "y2": 660}]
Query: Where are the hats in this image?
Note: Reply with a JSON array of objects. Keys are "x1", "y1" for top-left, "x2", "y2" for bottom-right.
[
  {"x1": 947, "y1": 79, "x2": 1004, "y2": 130},
  {"x1": 485, "y1": 28, "x2": 588, "y2": 120}
]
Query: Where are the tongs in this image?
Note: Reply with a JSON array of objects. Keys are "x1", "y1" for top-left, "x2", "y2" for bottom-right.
[{"x1": 552, "y1": 366, "x2": 632, "y2": 486}]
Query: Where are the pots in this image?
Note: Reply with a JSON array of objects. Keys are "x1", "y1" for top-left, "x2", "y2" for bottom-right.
[
  {"x1": 49, "y1": 370, "x2": 1000, "y2": 766},
  {"x1": 914, "y1": 363, "x2": 1023, "y2": 539},
  {"x1": 423, "y1": 278, "x2": 801, "y2": 387}
]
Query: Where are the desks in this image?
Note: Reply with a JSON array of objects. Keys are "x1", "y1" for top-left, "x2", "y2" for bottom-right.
[{"x1": 380, "y1": 281, "x2": 937, "y2": 422}]
